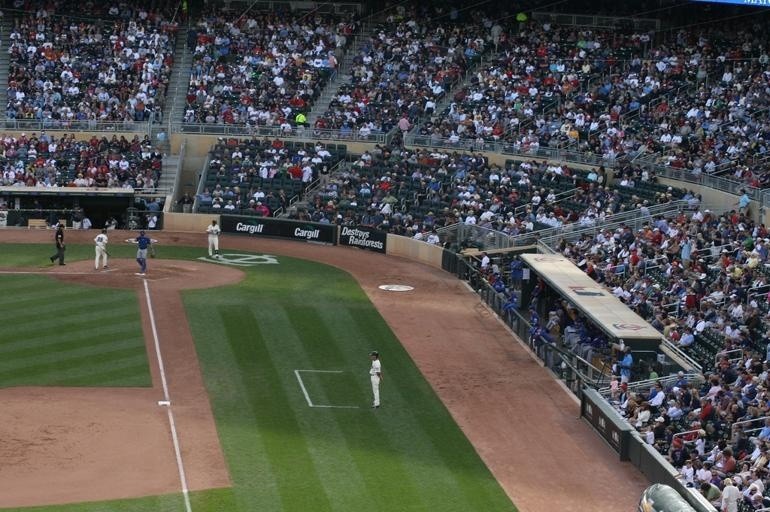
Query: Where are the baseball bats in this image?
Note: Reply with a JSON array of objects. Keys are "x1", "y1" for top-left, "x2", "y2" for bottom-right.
[{"x1": 98, "y1": 246, "x2": 111, "y2": 257}]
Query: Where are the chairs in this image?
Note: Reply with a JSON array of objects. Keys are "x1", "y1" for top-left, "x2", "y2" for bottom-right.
[{"x1": 199, "y1": 140, "x2": 683, "y2": 257}]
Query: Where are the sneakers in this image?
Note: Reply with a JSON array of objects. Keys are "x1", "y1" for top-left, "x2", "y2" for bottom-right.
[
  {"x1": 50, "y1": 257, "x2": 54, "y2": 263},
  {"x1": 59, "y1": 263, "x2": 66, "y2": 265},
  {"x1": 371, "y1": 401, "x2": 380, "y2": 408}
]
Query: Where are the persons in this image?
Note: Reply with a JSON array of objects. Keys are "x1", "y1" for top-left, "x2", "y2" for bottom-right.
[
  {"x1": 1, "y1": 0, "x2": 179, "y2": 131},
  {"x1": 196, "y1": 137, "x2": 702, "y2": 252},
  {"x1": 1, "y1": 197, "x2": 163, "y2": 230},
  {"x1": 472, "y1": 188, "x2": 770, "y2": 512},
  {"x1": 1, "y1": 131, "x2": 162, "y2": 193},
  {"x1": 50, "y1": 224, "x2": 66, "y2": 265},
  {"x1": 181, "y1": 1, "x2": 360, "y2": 135},
  {"x1": 135, "y1": 231, "x2": 153, "y2": 275},
  {"x1": 206, "y1": 220, "x2": 220, "y2": 258},
  {"x1": 369, "y1": 351, "x2": 382, "y2": 409},
  {"x1": 176, "y1": 193, "x2": 194, "y2": 213},
  {"x1": 314, "y1": 0, "x2": 770, "y2": 195},
  {"x1": 94, "y1": 231, "x2": 108, "y2": 269}
]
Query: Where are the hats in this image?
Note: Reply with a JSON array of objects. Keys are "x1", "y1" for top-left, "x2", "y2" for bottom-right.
[
  {"x1": 667, "y1": 398, "x2": 675, "y2": 404},
  {"x1": 705, "y1": 397, "x2": 712, "y2": 404},
  {"x1": 672, "y1": 248, "x2": 730, "y2": 266},
  {"x1": 653, "y1": 283, "x2": 661, "y2": 290},
  {"x1": 368, "y1": 351, "x2": 379, "y2": 356},
  {"x1": 745, "y1": 375, "x2": 753, "y2": 380},
  {"x1": 698, "y1": 428, "x2": 706, "y2": 437},
  {"x1": 427, "y1": 158, "x2": 675, "y2": 235},
  {"x1": 57, "y1": 222, "x2": 65, "y2": 227},
  {"x1": 215, "y1": 136, "x2": 263, "y2": 205},
  {"x1": 655, "y1": 416, "x2": 665, "y2": 423},
  {"x1": 3, "y1": 129, "x2": 85, "y2": 178},
  {"x1": 755, "y1": 385, "x2": 763, "y2": 390},
  {"x1": 619, "y1": 384, "x2": 627, "y2": 391}
]
